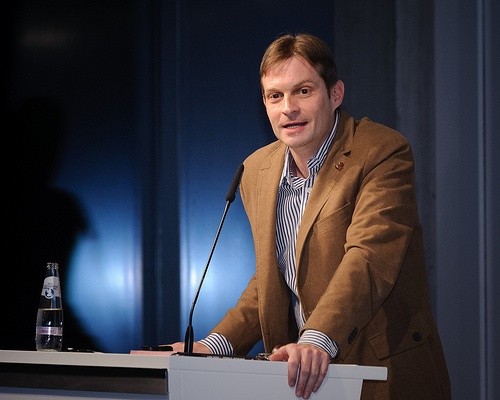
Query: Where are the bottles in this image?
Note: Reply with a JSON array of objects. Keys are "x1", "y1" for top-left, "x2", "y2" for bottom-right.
[{"x1": 36, "y1": 264, "x2": 63, "y2": 352}]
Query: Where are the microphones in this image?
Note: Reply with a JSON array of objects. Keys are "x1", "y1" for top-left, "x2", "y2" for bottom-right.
[{"x1": 184, "y1": 164, "x2": 243, "y2": 353}]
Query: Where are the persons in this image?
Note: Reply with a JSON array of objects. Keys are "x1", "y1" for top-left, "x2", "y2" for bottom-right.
[{"x1": 132, "y1": 32, "x2": 452, "y2": 400}]
